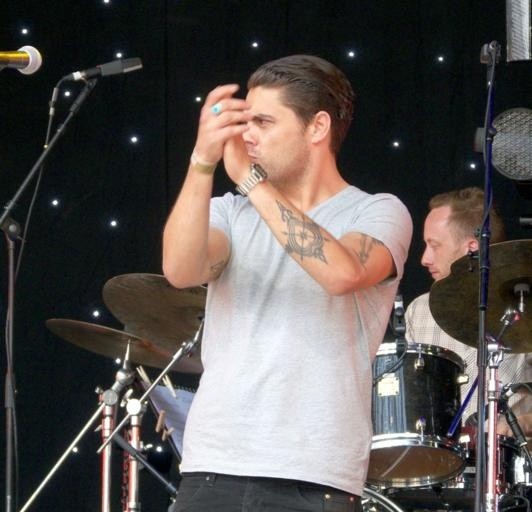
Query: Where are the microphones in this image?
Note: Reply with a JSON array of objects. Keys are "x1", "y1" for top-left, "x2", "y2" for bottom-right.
[
  {"x1": 480, "y1": 41, "x2": 502, "y2": 65},
  {"x1": 63, "y1": 57, "x2": 143, "y2": 83},
  {"x1": 0, "y1": 45, "x2": 43, "y2": 75}
]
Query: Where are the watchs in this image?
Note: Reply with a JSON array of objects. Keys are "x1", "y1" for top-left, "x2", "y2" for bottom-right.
[{"x1": 235, "y1": 164, "x2": 267, "y2": 197}]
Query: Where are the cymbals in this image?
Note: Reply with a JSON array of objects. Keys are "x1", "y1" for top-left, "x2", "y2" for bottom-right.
[
  {"x1": 428, "y1": 240, "x2": 531, "y2": 355},
  {"x1": 45, "y1": 317, "x2": 203, "y2": 374},
  {"x1": 103, "y1": 274, "x2": 206, "y2": 368}
]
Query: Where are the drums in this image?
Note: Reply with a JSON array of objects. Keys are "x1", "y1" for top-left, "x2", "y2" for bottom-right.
[
  {"x1": 365, "y1": 341, "x2": 468, "y2": 489},
  {"x1": 388, "y1": 433, "x2": 531, "y2": 498},
  {"x1": 331, "y1": 485, "x2": 463, "y2": 511}
]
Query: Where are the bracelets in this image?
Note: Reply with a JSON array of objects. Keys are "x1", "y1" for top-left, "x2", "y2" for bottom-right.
[{"x1": 190, "y1": 149, "x2": 217, "y2": 175}]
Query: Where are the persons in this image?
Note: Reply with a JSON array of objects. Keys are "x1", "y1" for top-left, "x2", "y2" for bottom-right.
[
  {"x1": 404, "y1": 187, "x2": 532, "y2": 451},
  {"x1": 162, "y1": 54, "x2": 414, "y2": 512}
]
